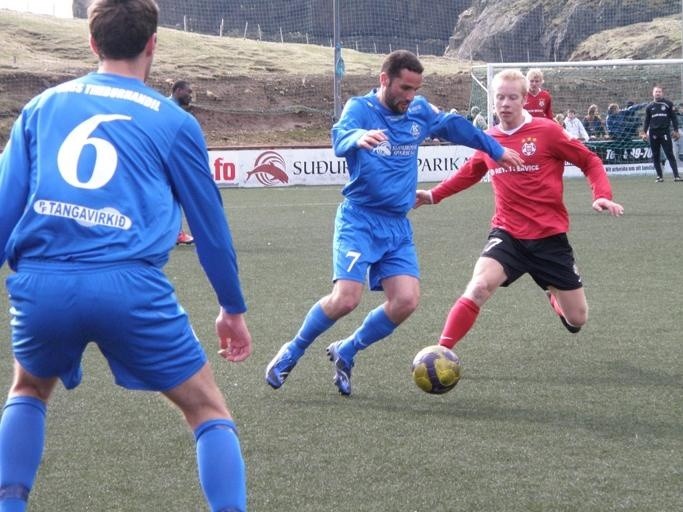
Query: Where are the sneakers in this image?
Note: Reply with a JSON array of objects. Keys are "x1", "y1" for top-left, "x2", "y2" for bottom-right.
[
  {"x1": 177, "y1": 233, "x2": 193, "y2": 249},
  {"x1": 674, "y1": 176, "x2": 681, "y2": 184},
  {"x1": 654, "y1": 176, "x2": 664, "y2": 184},
  {"x1": 547, "y1": 293, "x2": 584, "y2": 334},
  {"x1": 325, "y1": 339, "x2": 356, "y2": 397},
  {"x1": 266, "y1": 343, "x2": 299, "y2": 390}
]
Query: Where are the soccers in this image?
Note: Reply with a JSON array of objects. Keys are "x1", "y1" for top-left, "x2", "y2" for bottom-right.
[{"x1": 411, "y1": 345, "x2": 462, "y2": 395}]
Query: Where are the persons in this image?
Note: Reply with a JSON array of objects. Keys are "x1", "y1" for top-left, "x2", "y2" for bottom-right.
[
  {"x1": 413, "y1": 68, "x2": 625, "y2": 349},
  {"x1": 467, "y1": 106, "x2": 480, "y2": 120},
  {"x1": 581, "y1": 104, "x2": 609, "y2": 140},
  {"x1": 265, "y1": 49, "x2": 525, "y2": 396},
  {"x1": 0, "y1": 1, "x2": 252, "y2": 512},
  {"x1": 164, "y1": 81, "x2": 194, "y2": 245},
  {"x1": 605, "y1": 103, "x2": 649, "y2": 160},
  {"x1": 472, "y1": 113, "x2": 487, "y2": 129},
  {"x1": 642, "y1": 87, "x2": 683, "y2": 182},
  {"x1": 450, "y1": 108, "x2": 457, "y2": 114},
  {"x1": 674, "y1": 103, "x2": 683, "y2": 161},
  {"x1": 554, "y1": 113, "x2": 564, "y2": 126},
  {"x1": 522, "y1": 69, "x2": 553, "y2": 120},
  {"x1": 625, "y1": 102, "x2": 640, "y2": 159},
  {"x1": 563, "y1": 110, "x2": 589, "y2": 142}
]
